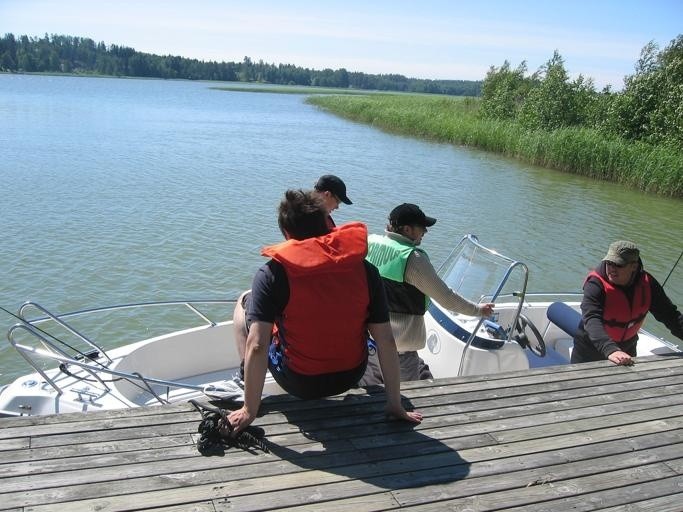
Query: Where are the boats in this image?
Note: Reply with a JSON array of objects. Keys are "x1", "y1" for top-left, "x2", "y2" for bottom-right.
[{"x1": 0, "y1": 233, "x2": 683, "y2": 420}]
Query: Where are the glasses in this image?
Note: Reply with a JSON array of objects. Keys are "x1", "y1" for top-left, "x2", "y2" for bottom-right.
[{"x1": 604, "y1": 261, "x2": 626, "y2": 268}]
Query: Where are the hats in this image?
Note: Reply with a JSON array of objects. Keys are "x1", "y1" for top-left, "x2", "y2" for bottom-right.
[
  {"x1": 601, "y1": 240, "x2": 639, "y2": 267},
  {"x1": 388, "y1": 202, "x2": 437, "y2": 228},
  {"x1": 316, "y1": 175, "x2": 354, "y2": 205}
]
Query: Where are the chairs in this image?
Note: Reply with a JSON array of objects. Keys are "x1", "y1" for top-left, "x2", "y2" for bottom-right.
[{"x1": 523, "y1": 303, "x2": 583, "y2": 369}]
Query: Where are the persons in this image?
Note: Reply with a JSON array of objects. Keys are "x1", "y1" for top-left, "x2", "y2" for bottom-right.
[
  {"x1": 355, "y1": 203, "x2": 495, "y2": 387},
  {"x1": 571, "y1": 240, "x2": 682, "y2": 366},
  {"x1": 312, "y1": 173, "x2": 353, "y2": 228},
  {"x1": 216, "y1": 190, "x2": 424, "y2": 437}
]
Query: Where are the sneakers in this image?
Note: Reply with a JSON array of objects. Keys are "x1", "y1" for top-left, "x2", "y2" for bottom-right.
[{"x1": 203, "y1": 377, "x2": 245, "y2": 403}]
